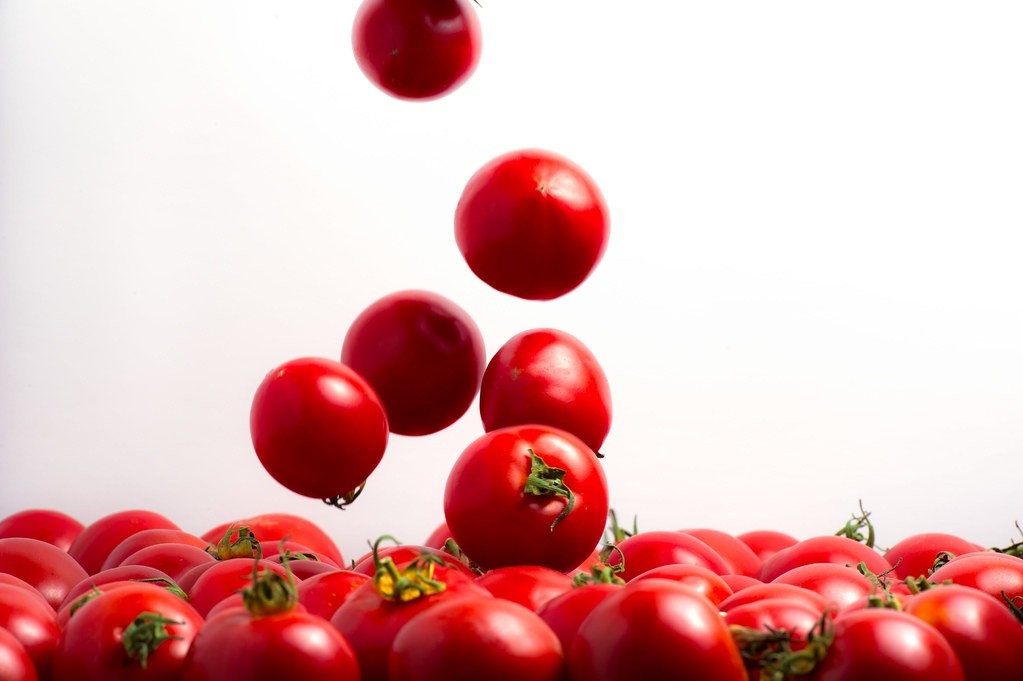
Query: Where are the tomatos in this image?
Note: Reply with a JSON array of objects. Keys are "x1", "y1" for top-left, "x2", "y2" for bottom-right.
[{"x1": 0, "y1": 2, "x2": 1023, "y2": 681}]
